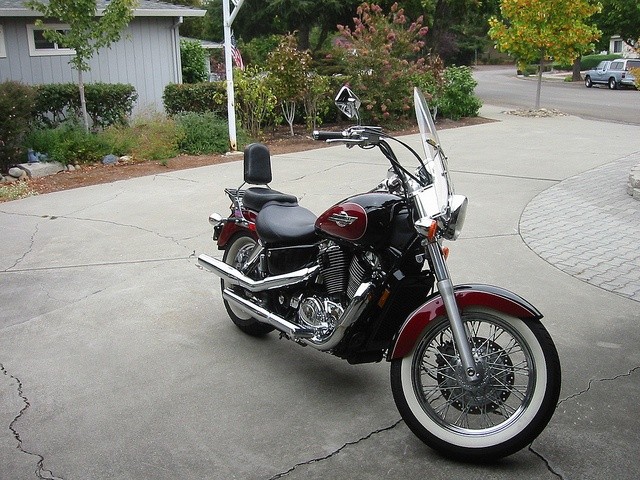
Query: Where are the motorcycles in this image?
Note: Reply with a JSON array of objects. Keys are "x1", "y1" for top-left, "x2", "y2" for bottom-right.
[{"x1": 198, "y1": 86, "x2": 562, "y2": 463}]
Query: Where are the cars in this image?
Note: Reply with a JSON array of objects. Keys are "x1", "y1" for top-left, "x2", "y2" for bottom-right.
[{"x1": 585, "y1": 59, "x2": 639, "y2": 90}]
